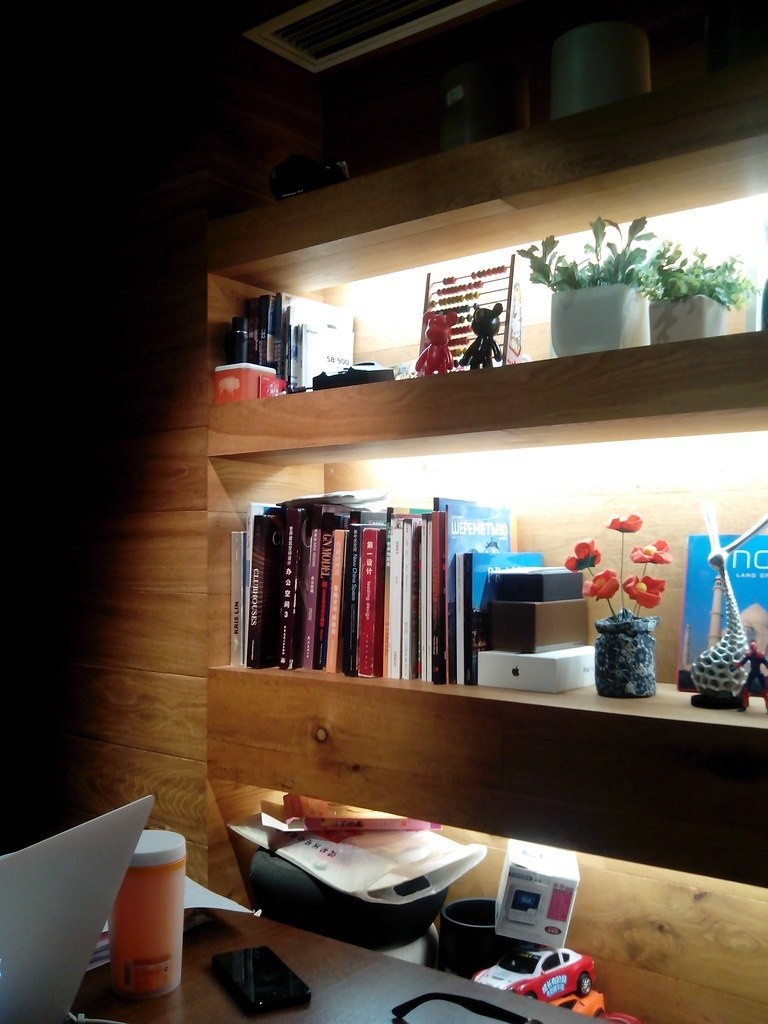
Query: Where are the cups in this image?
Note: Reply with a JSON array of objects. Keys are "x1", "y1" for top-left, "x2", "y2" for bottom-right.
[
  {"x1": 438, "y1": 898, "x2": 535, "y2": 980},
  {"x1": 110, "y1": 829, "x2": 186, "y2": 997}
]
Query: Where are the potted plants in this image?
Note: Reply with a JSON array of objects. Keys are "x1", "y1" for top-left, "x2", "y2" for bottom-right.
[{"x1": 516, "y1": 219, "x2": 759, "y2": 357}]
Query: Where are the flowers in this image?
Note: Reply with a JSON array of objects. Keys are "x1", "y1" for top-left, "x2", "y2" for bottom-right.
[{"x1": 566, "y1": 516, "x2": 674, "y2": 620}]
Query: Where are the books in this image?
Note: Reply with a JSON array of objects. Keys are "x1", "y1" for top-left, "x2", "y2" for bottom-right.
[
  {"x1": 230, "y1": 292, "x2": 354, "y2": 394},
  {"x1": 229, "y1": 488, "x2": 543, "y2": 687}
]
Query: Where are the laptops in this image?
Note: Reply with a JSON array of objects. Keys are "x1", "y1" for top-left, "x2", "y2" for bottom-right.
[{"x1": 0, "y1": 797, "x2": 157, "y2": 1024}]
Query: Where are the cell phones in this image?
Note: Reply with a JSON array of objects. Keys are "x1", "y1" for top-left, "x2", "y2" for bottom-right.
[{"x1": 212, "y1": 946, "x2": 312, "y2": 1017}]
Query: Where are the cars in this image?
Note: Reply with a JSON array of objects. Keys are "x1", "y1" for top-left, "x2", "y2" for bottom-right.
[
  {"x1": 598, "y1": 1011, "x2": 644, "y2": 1024},
  {"x1": 472, "y1": 946, "x2": 596, "y2": 1002},
  {"x1": 548, "y1": 988, "x2": 605, "y2": 1018}
]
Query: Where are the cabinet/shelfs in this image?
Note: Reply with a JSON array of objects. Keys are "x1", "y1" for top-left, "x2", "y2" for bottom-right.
[{"x1": 183, "y1": 62, "x2": 768, "y2": 1024}]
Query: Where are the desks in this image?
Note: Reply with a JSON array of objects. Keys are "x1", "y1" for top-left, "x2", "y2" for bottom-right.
[{"x1": 66, "y1": 909, "x2": 611, "y2": 1024}]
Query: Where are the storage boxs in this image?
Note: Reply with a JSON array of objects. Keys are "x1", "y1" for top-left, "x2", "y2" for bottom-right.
[
  {"x1": 215, "y1": 363, "x2": 276, "y2": 404},
  {"x1": 477, "y1": 566, "x2": 593, "y2": 694},
  {"x1": 493, "y1": 839, "x2": 581, "y2": 952},
  {"x1": 260, "y1": 792, "x2": 445, "y2": 832}
]
